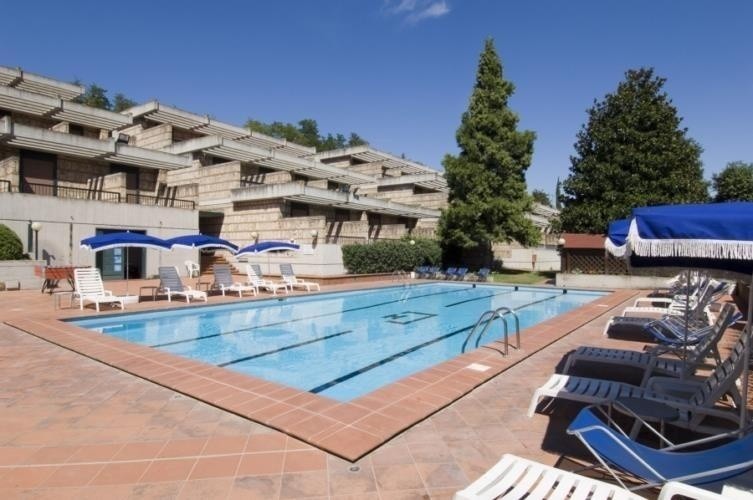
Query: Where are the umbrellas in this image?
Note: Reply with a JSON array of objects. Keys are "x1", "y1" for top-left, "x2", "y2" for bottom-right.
[
  {"x1": 234, "y1": 241, "x2": 300, "y2": 281},
  {"x1": 81, "y1": 230, "x2": 172, "y2": 296},
  {"x1": 604, "y1": 200, "x2": 753, "y2": 439},
  {"x1": 168, "y1": 232, "x2": 240, "y2": 290}
]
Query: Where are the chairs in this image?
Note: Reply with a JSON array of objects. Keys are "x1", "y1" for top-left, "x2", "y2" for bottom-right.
[
  {"x1": 453, "y1": 453, "x2": 753, "y2": 499},
  {"x1": 566, "y1": 399, "x2": 752, "y2": 492},
  {"x1": 526, "y1": 321, "x2": 753, "y2": 439},
  {"x1": 156, "y1": 265, "x2": 322, "y2": 303},
  {"x1": 560, "y1": 273, "x2": 752, "y2": 395},
  {"x1": 71, "y1": 266, "x2": 123, "y2": 313}
]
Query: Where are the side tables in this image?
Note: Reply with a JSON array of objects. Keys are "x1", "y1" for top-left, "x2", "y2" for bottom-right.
[
  {"x1": 54, "y1": 290, "x2": 73, "y2": 311},
  {"x1": 139, "y1": 285, "x2": 156, "y2": 301}
]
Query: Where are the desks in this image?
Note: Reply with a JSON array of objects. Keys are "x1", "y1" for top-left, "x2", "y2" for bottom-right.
[{"x1": 612, "y1": 397, "x2": 680, "y2": 451}]
